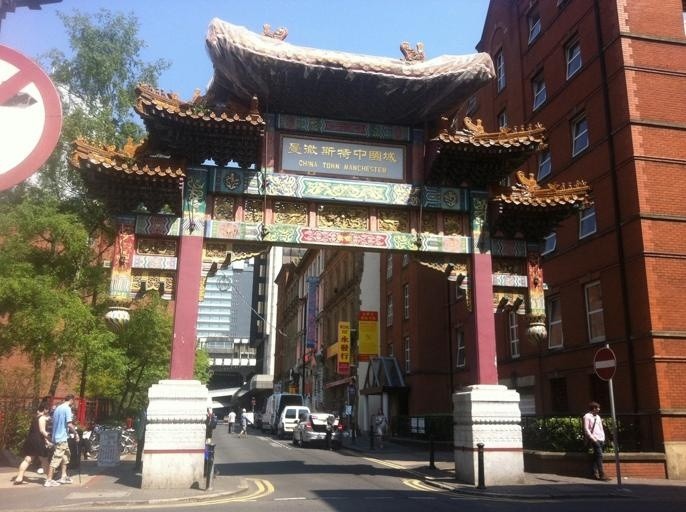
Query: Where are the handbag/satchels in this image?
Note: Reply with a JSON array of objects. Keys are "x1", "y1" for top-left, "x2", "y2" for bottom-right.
[{"x1": 583, "y1": 439, "x2": 592, "y2": 451}]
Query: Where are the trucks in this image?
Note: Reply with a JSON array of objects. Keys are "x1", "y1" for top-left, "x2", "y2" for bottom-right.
[{"x1": 261, "y1": 393, "x2": 304, "y2": 435}]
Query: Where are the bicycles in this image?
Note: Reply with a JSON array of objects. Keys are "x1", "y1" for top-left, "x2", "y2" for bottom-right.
[{"x1": 86, "y1": 421, "x2": 138, "y2": 461}]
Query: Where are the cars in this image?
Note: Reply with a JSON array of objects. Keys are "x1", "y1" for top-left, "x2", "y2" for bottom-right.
[{"x1": 291, "y1": 411, "x2": 349, "y2": 450}]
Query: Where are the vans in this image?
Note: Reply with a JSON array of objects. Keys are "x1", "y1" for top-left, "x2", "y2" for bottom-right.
[{"x1": 276, "y1": 405, "x2": 311, "y2": 440}]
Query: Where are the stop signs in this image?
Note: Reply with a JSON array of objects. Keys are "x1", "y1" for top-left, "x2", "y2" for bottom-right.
[{"x1": 593, "y1": 343, "x2": 622, "y2": 489}]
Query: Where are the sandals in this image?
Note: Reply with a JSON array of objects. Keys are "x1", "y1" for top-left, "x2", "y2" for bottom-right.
[{"x1": 14, "y1": 479, "x2": 28, "y2": 485}]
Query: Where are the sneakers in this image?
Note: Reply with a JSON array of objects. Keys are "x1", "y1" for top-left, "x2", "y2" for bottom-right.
[
  {"x1": 44, "y1": 479, "x2": 61, "y2": 486},
  {"x1": 59, "y1": 475, "x2": 73, "y2": 483}
]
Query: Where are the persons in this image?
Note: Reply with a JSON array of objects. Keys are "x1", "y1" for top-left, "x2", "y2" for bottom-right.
[
  {"x1": 583, "y1": 401, "x2": 611, "y2": 481},
  {"x1": 44, "y1": 394, "x2": 80, "y2": 487},
  {"x1": 375, "y1": 408, "x2": 388, "y2": 448},
  {"x1": 13, "y1": 401, "x2": 52, "y2": 485},
  {"x1": 228, "y1": 408, "x2": 237, "y2": 434},
  {"x1": 325, "y1": 416, "x2": 334, "y2": 451},
  {"x1": 237, "y1": 408, "x2": 248, "y2": 439}
]
